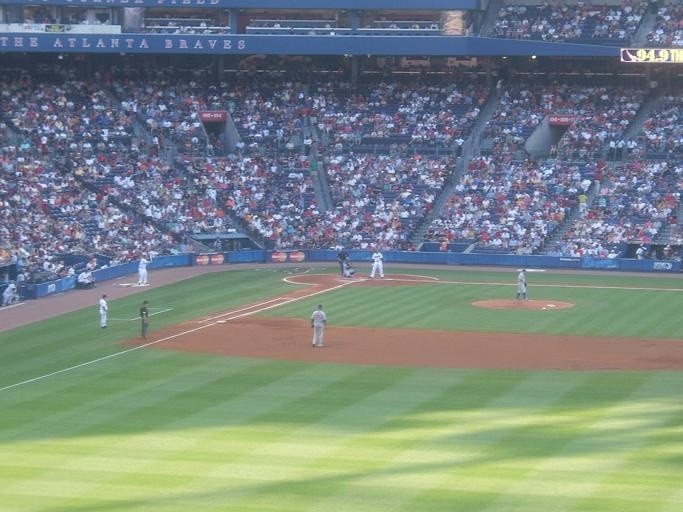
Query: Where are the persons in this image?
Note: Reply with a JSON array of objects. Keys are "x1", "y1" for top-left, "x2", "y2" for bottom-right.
[
  {"x1": 140, "y1": 300, "x2": 149, "y2": 339},
  {"x1": 99, "y1": 294, "x2": 109, "y2": 328},
  {"x1": 2, "y1": 283, "x2": 17, "y2": 306},
  {"x1": 515, "y1": 269, "x2": 529, "y2": 302},
  {"x1": 311, "y1": 304, "x2": 329, "y2": 348}
]
